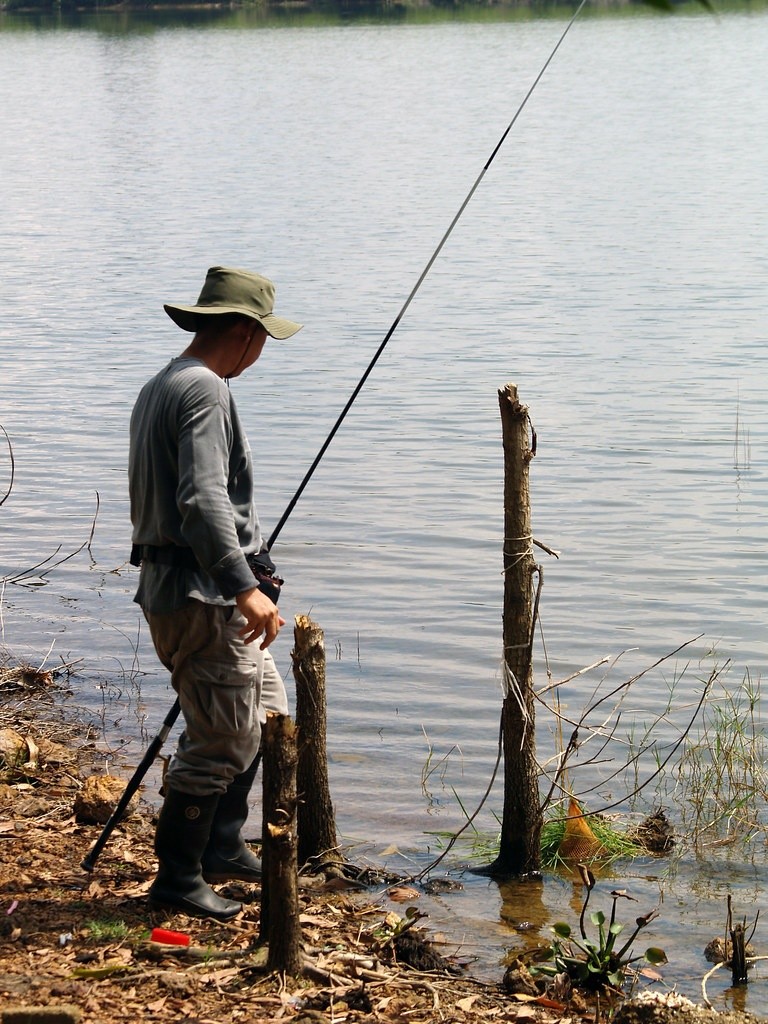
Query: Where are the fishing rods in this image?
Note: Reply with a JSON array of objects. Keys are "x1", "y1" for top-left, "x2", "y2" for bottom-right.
[{"x1": 80, "y1": 0, "x2": 586, "y2": 873}]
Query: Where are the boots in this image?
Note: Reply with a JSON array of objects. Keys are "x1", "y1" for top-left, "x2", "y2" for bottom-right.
[
  {"x1": 201, "y1": 786, "x2": 263, "y2": 881},
  {"x1": 147, "y1": 790, "x2": 243, "y2": 921}
]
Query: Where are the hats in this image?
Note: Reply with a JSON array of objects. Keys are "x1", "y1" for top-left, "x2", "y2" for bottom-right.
[{"x1": 163, "y1": 267, "x2": 304, "y2": 340}]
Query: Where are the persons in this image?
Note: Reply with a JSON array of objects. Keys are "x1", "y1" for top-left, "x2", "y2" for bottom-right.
[{"x1": 124, "y1": 262, "x2": 306, "y2": 917}]
